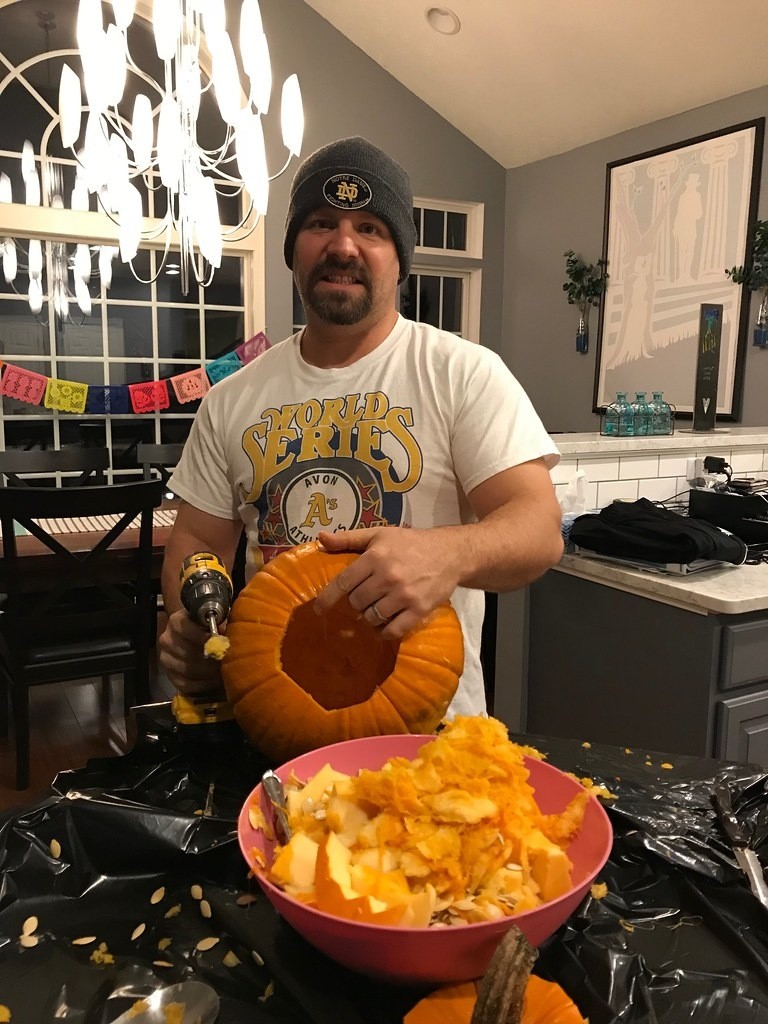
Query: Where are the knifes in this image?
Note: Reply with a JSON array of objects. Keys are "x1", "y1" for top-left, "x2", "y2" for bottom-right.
[{"x1": 712, "y1": 785, "x2": 768, "y2": 906}]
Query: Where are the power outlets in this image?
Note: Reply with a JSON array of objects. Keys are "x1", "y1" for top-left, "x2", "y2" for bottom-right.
[{"x1": 687, "y1": 457, "x2": 717, "y2": 480}]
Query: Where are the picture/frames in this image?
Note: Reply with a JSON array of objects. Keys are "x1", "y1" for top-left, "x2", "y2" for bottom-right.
[{"x1": 592, "y1": 116, "x2": 765, "y2": 422}]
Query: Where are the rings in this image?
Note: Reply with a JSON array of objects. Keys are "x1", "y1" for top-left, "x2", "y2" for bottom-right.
[{"x1": 368, "y1": 602, "x2": 388, "y2": 624}]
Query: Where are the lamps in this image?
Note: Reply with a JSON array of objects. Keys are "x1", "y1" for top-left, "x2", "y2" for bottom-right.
[
  {"x1": 58, "y1": 0, "x2": 309, "y2": 294},
  {"x1": 0, "y1": 141, "x2": 122, "y2": 328}
]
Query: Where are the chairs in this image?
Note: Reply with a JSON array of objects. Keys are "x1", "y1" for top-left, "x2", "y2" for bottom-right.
[
  {"x1": 136, "y1": 442, "x2": 186, "y2": 482},
  {"x1": 0, "y1": 480, "x2": 164, "y2": 791},
  {"x1": 0, "y1": 448, "x2": 110, "y2": 488}
]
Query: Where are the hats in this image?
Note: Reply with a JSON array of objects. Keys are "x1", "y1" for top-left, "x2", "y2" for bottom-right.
[{"x1": 282, "y1": 136, "x2": 416, "y2": 283}]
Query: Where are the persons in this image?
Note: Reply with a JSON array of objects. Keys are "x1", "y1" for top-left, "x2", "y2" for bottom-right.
[{"x1": 157, "y1": 137, "x2": 566, "y2": 732}]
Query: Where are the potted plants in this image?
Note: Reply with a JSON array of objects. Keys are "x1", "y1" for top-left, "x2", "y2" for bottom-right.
[
  {"x1": 725, "y1": 218, "x2": 768, "y2": 349},
  {"x1": 562, "y1": 248, "x2": 609, "y2": 353}
]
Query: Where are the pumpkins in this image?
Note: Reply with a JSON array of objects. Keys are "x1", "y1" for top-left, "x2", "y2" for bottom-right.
[{"x1": 219, "y1": 540, "x2": 466, "y2": 761}]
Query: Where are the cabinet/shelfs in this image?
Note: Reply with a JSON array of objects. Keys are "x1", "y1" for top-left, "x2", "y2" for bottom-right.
[{"x1": 527, "y1": 565, "x2": 768, "y2": 769}]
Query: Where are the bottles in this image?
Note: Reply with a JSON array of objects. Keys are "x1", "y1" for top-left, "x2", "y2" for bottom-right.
[
  {"x1": 605, "y1": 392, "x2": 633, "y2": 436},
  {"x1": 631, "y1": 393, "x2": 653, "y2": 436},
  {"x1": 649, "y1": 392, "x2": 672, "y2": 434}
]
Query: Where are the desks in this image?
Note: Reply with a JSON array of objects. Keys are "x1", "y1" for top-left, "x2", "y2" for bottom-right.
[
  {"x1": 0, "y1": 509, "x2": 182, "y2": 617},
  {"x1": 0, "y1": 728, "x2": 768, "y2": 1024}
]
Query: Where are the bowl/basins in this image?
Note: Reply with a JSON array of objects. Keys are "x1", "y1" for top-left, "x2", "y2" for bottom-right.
[{"x1": 238, "y1": 734, "x2": 614, "y2": 984}]
